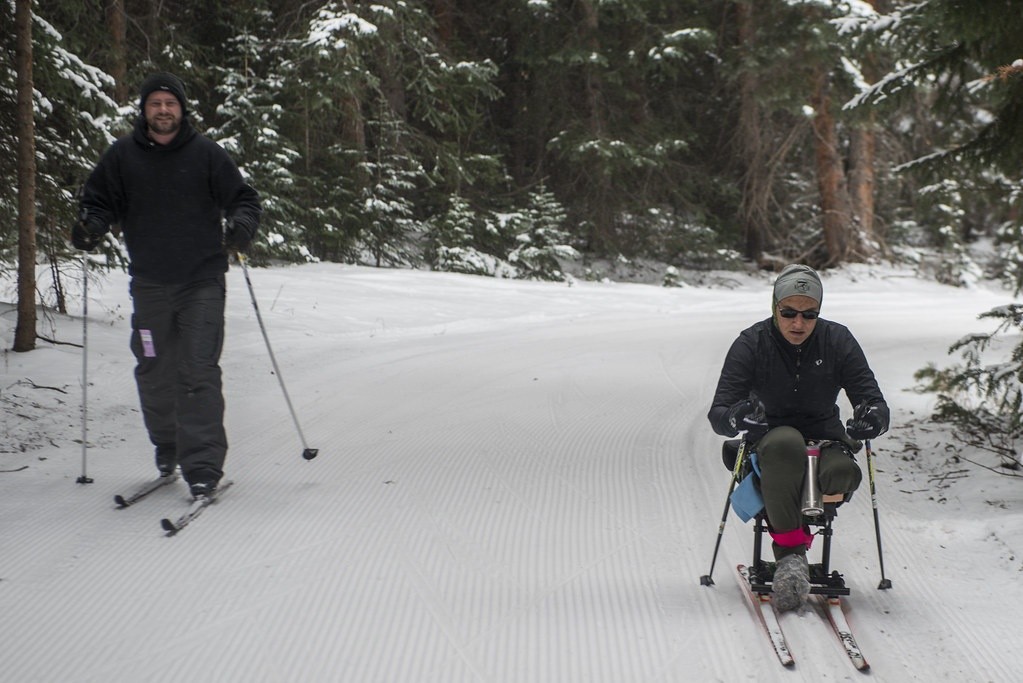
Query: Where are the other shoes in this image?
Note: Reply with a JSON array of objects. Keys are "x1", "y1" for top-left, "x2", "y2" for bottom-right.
[
  {"x1": 156, "y1": 445, "x2": 176, "y2": 471},
  {"x1": 772, "y1": 553, "x2": 810, "y2": 612},
  {"x1": 189, "y1": 471, "x2": 219, "y2": 494}
]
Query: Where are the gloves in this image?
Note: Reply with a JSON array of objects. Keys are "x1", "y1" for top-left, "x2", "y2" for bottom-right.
[
  {"x1": 71, "y1": 218, "x2": 104, "y2": 251},
  {"x1": 216, "y1": 219, "x2": 251, "y2": 253},
  {"x1": 846, "y1": 405, "x2": 881, "y2": 439},
  {"x1": 719, "y1": 398, "x2": 769, "y2": 442}
]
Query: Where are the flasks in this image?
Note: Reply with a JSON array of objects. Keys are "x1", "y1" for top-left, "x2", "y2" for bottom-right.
[{"x1": 802, "y1": 446, "x2": 824, "y2": 517}]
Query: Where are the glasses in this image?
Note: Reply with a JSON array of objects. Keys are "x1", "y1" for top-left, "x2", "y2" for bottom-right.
[{"x1": 774, "y1": 296, "x2": 822, "y2": 319}]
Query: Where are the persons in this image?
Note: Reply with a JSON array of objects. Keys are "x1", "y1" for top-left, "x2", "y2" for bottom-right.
[
  {"x1": 708, "y1": 264, "x2": 889, "y2": 610},
  {"x1": 70, "y1": 72, "x2": 262, "y2": 499}
]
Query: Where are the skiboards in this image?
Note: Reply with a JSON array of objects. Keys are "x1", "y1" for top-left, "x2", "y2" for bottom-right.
[
  {"x1": 113, "y1": 466, "x2": 236, "y2": 533},
  {"x1": 736, "y1": 563, "x2": 872, "y2": 672}
]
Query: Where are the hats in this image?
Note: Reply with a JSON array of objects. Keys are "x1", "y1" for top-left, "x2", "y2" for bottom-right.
[
  {"x1": 772, "y1": 264, "x2": 823, "y2": 313},
  {"x1": 140, "y1": 71, "x2": 187, "y2": 117}
]
